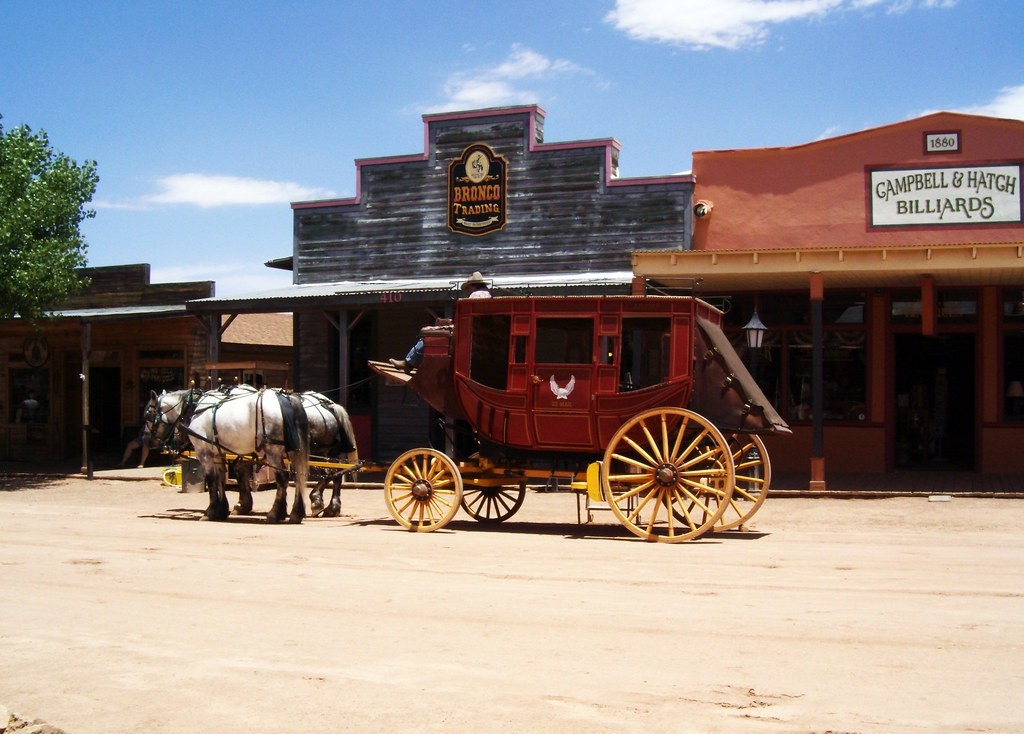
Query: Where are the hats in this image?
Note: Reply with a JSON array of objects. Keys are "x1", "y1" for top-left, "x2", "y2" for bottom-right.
[{"x1": 461, "y1": 271, "x2": 491, "y2": 291}]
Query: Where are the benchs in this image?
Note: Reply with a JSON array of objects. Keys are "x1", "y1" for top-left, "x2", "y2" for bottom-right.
[{"x1": 421, "y1": 324, "x2": 453, "y2": 360}]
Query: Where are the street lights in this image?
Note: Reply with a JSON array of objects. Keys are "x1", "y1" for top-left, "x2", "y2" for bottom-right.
[{"x1": 741, "y1": 306, "x2": 769, "y2": 493}]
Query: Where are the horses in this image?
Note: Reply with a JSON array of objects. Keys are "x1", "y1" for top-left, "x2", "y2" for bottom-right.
[
  {"x1": 204, "y1": 384, "x2": 359, "y2": 518},
  {"x1": 143, "y1": 388, "x2": 309, "y2": 525}
]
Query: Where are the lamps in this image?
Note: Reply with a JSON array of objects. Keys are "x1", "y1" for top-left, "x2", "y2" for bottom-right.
[
  {"x1": 1007, "y1": 382, "x2": 1024, "y2": 418},
  {"x1": 740, "y1": 305, "x2": 769, "y2": 354}
]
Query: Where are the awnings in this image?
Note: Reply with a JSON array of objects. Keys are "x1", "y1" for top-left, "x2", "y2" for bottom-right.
[
  {"x1": 187, "y1": 268, "x2": 633, "y2": 336},
  {"x1": 1, "y1": 304, "x2": 194, "y2": 324}
]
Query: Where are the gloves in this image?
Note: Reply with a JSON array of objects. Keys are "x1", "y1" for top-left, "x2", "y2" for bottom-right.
[{"x1": 435, "y1": 317, "x2": 452, "y2": 326}]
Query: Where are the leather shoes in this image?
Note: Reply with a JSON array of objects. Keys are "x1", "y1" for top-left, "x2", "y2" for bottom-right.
[{"x1": 388, "y1": 358, "x2": 413, "y2": 374}]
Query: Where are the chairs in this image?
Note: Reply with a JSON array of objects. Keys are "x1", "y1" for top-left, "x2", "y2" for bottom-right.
[{"x1": 119, "y1": 419, "x2": 173, "y2": 467}]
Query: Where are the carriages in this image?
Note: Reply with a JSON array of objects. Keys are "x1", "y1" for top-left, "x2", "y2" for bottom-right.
[{"x1": 140, "y1": 281, "x2": 795, "y2": 544}]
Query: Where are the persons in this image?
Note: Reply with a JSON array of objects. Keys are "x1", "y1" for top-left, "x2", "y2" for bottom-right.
[{"x1": 390, "y1": 272, "x2": 492, "y2": 373}]
